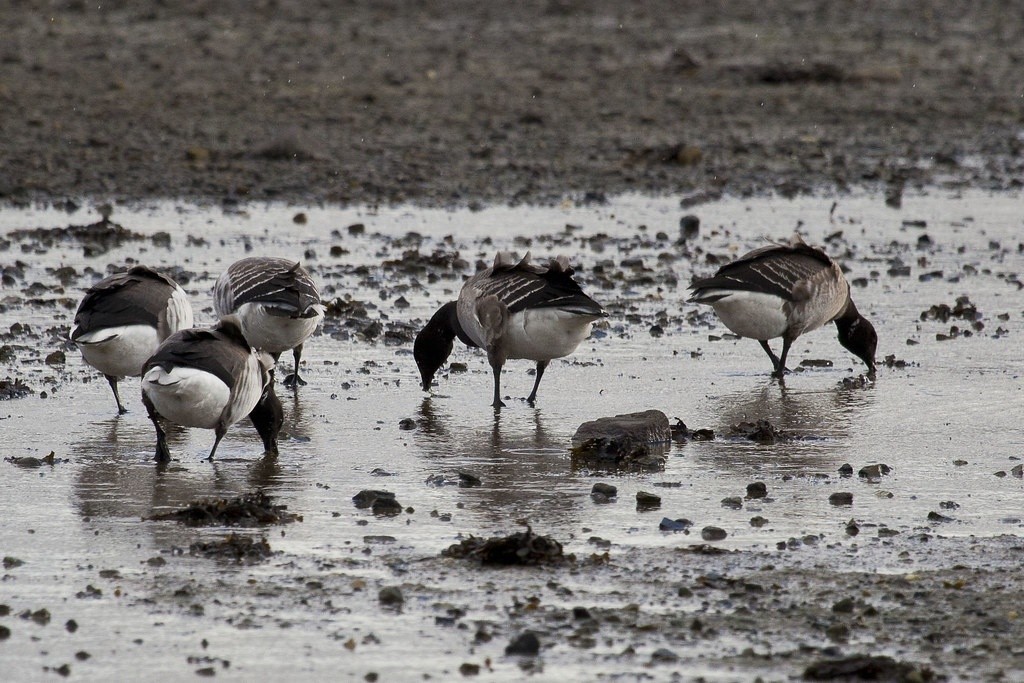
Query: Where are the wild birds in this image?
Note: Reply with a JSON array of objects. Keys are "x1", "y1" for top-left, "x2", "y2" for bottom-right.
[
  {"x1": 141, "y1": 327, "x2": 285, "y2": 464},
  {"x1": 413, "y1": 251, "x2": 611, "y2": 409},
  {"x1": 686, "y1": 232, "x2": 878, "y2": 379},
  {"x1": 212, "y1": 256, "x2": 322, "y2": 390},
  {"x1": 68, "y1": 263, "x2": 195, "y2": 415}
]
